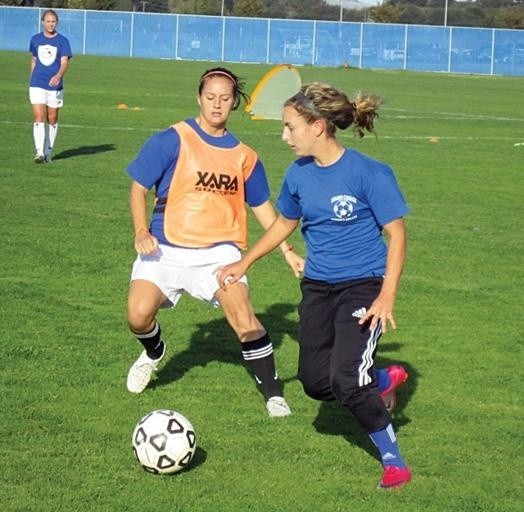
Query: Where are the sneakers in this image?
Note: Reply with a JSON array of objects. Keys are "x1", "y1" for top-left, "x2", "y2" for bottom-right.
[
  {"x1": 127, "y1": 340, "x2": 166, "y2": 393},
  {"x1": 267, "y1": 396, "x2": 291, "y2": 417},
  {"x1": 34, "y1": 156, "x2": 51, "y2": 163},
  {"x1": 377, "y1": 466, "x2": 411, "y2": 490},
  {"x1": 379, "y1": 366, "x2": 408, "y2": 412}
]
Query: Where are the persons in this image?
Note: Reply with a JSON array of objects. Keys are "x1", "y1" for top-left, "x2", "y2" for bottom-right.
[
  {"x1": 211, "y1": 81, "x2": 411, "y2": 492},
  {"x1": 28, "y1": 10, "x2": 72, "y2": 164},
  {"x1": 125, "y1": 67, "x2": 306, "y2": 417}
]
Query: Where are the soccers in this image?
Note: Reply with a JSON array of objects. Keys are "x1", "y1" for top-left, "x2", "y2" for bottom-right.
[{"x1": 132, "y1": 409, "x2": 195, "y2": 474}]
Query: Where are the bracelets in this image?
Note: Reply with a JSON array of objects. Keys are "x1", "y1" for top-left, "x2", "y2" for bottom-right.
[{"x1": 282, "y1": 245, "x2": 295, "y2": 255}]
